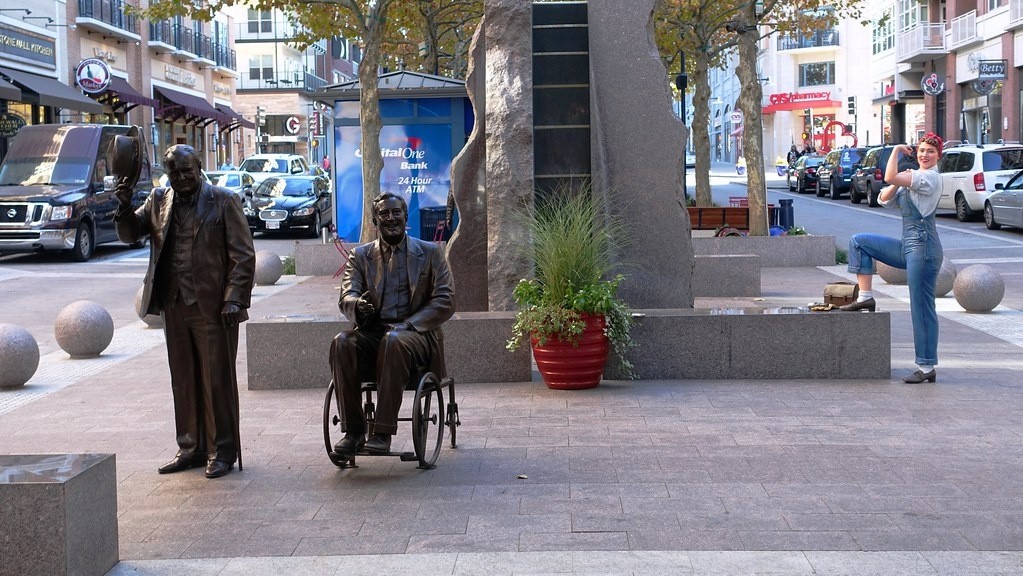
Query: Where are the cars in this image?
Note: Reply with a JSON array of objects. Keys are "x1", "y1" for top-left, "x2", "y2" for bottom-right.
[
  {"x1": 159, "y1": 154, "x2": 333, "y2": 237},
  {"x1": 686, "y1": 151, "x2": 696, "y2": 167},
  {"x1": 788, "y1": 154, "x2": 825, "y2": 194},
  {"x1": 984, "y1": 170, "x2": 1023, "y2": 230}
]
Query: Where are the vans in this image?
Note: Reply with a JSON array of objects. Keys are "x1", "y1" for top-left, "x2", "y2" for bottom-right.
[{"x1": 0, "y1": 122, "x2": 154, "y2": 261}]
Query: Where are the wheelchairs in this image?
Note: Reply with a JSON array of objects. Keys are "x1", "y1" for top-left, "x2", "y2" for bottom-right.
[{"x1": 322, "y1": 372, "x2": 460, "y2": 469}]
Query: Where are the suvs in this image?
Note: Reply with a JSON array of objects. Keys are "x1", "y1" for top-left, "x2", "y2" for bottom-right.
[
  {"x1": 935, "y1": 140, "x2": 1023, "y2": 221},
  {"x1": 814, "y1": 142, "x2": 919, "y2": 208}
]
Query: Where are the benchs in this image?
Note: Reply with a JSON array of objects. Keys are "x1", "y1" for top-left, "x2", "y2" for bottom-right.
[
  {"x1": 682, "y1": 205, "x2": 782, "y2": 232},
  {"x1": 729, "y1": 196, "x2": 748, "y2": 206}
]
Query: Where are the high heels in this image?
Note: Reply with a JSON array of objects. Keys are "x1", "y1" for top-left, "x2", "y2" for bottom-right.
[
  {"x1": 902, "y1": 368, "x2": 936, "y2": 384},
  {"x1": 839, "y1": 296, "x2": 876, "y2": 313}
]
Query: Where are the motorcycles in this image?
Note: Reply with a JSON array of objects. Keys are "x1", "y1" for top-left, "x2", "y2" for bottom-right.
[
  {"x1": 736, "y1": 156, "x2": 748, "y2": 175},
  {"x1": 775, "y1": 155, "x2": 788, "y2": 176}
]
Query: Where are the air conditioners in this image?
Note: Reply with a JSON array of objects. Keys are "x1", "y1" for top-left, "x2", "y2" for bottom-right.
[{"x1": 262, "y1": 135, "x2": 270, "y2": 143}]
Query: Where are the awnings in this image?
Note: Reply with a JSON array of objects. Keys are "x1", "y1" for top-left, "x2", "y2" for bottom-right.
[
  {"x1": 105, "y1": 75, "x2": 158, "y2": 113},
  {"x1": 154, "y1": 85, "x2": 232, "y2": 127},
  {"x1": 0, "y1": 65, "x2": 103, "y2": 115},
  {"x1": 215, "y1": 102, "x2": 255, "y2": 129}
]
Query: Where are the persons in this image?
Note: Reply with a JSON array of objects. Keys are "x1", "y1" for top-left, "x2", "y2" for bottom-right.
[
  {"x1": 840, "y1": 131, "x2": 944, "y2": 383},
  {"x1": 788, "y1": 144, "x2": 816, "y2": 166},
  {"x1": 114, "y1": 144, "x2": 255, "y2": 478},
  {"x1": 329, "y1": 192, "x2": 455, "y2": 455},
  {"x1": 323, "y1": 155, "x2": 329, "y2": 172},
  {"x1": 220, "y1": 159, "x2": 236, "y2": 170}
]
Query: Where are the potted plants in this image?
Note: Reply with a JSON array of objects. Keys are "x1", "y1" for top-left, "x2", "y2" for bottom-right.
[
  {"x1": 467, "y1": 170, "x2": 638, "y2": 390},
  {"x1": 736, "y1": 156, "x2": 746, "y2": 175},
  {"x1": 775, "y1": 155, "x2": 788, "y2": 176}
]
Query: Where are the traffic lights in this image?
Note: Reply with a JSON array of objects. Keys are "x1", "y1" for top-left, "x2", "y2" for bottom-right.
[
  {"x1": 312, "y1": 139, "x2": 319, "y2": 147},
  {"x1": 802, "y1": 133, "x2": 810, "y2": 141},
  {"x1": 849, "y1": 96, "x2": 857, "y2": 115},
  {"x1": 805, "y1": 109, "x2": 813, "y2": 123},
  {"x1": 258, "y1": 108, "x2": 266, "y2": 126},
  {"x1": 309, "y1": 116, "x2": 316, "y2": 133}
]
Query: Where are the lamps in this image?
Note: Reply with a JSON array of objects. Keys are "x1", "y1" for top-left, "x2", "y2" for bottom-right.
[
  {"x1": 0, "y1": 7, "x2": 32, "y2": 14},
  {"x1": 23, "y1": 15, "x2": 54, "y2": 23},
  {"x1": 45, "y1": 23, "x2": 77, "y2": 32},
  {"x1": 401, "y1": 62, "x2": 407, "y2": 71}
]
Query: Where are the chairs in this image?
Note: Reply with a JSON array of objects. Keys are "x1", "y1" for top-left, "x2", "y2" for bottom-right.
[
  {"x1": 929, "y1": 35, "x2": 943, "y2": 46},
  {"x1": 330, "y1": 223, "x2": 350, "y2": 278},
  {"x1": 434, "y1": 219, "x2": 446, "y2": 243}
]
